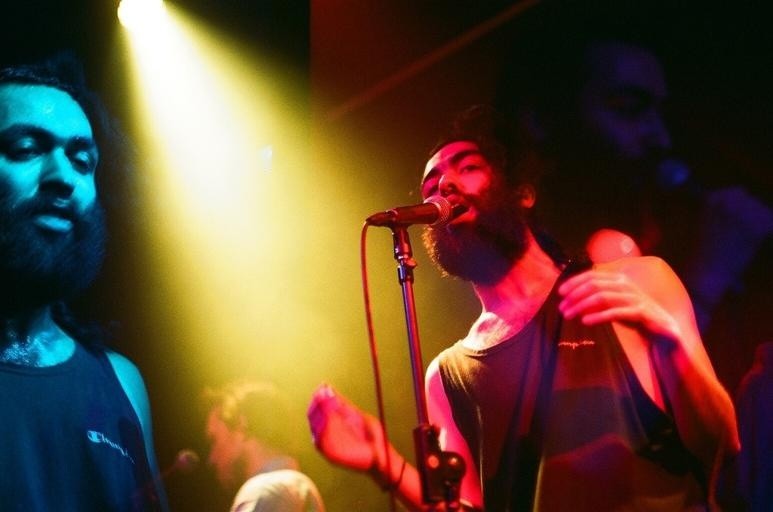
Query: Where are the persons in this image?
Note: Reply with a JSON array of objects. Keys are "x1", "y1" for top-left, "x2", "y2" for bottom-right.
[
  {"x1": 708, "y1": 342, "x2": 773, "y2": 512},
  {"x1": 200, "y1": 375, "x2": 328, "y2": 512},
  {"x1": 0, "y1": 66, "x2": 171, "y2": 512},
  {"x1": 308, "y1": 134, "x2": 742, "y2": 512}
]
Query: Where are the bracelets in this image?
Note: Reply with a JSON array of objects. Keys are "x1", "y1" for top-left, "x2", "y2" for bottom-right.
[{"x1": 395, "y1": 456, "x2": 406, "y2": 492}]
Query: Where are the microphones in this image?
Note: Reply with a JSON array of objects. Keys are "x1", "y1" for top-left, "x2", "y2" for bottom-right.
[
  {"x1": 159, "y1": 449, "x2": 199, "y2": 481},
  {"x1": 366, "y1": 194, "x2": 453, "y2": 228}
]
